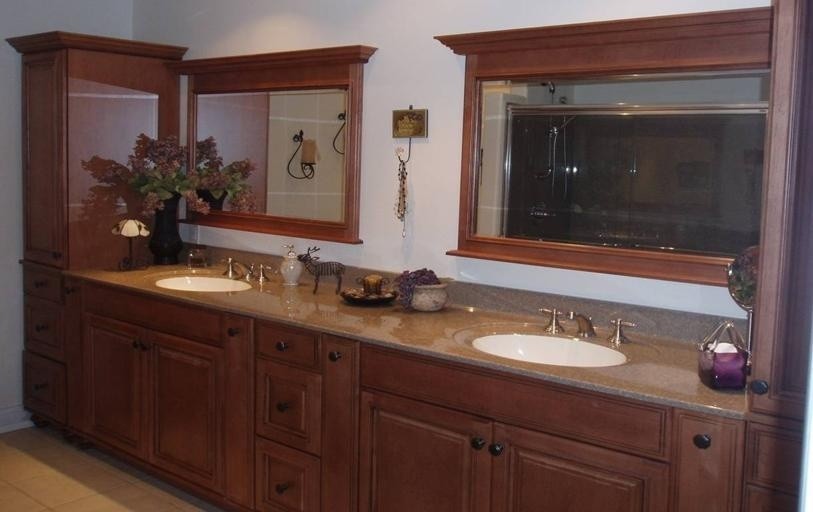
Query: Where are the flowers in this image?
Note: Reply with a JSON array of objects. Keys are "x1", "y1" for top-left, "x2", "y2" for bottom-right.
[
  {"x1": 190, "y1": 135, "x2": 260, "y2": 216},
  {"x1": 75, "y1": 128, "x2": 236, "y2": 218}
]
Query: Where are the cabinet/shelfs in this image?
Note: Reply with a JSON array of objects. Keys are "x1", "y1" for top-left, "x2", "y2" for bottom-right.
[
  {"x1": 676, "y1": 408, "x2": 743, "y2": 511},
  {"x1": 743, "y1": 409, "x2": 804, "y2": 511},
  {"x1": 5, "y1": 28, "x2": 188, "y2": 271},
  {"x1": 253, "y1": 315, "x2": 322, "y2": 511},
  {"x1": 499, "y1": 99, "x2": 769, "y2": 259},
  {"x1": 356, "y1": 336, "x2": 675, "y2": 511},
  {"x1": 69, "y1": 274, "x2": 223, "y2": 512},
  {"x1": 223, "y1": 307, "x2": 254, "y2": 510},
  {"x1": 18, "y1": 256, "x2": 68, "y2": 442},
  {"x1": 322, "y1": 331, "x2": 356, "y2": 512},
  {"x1": 742, "y1": 0, "x2": 811, "y2": 422}
]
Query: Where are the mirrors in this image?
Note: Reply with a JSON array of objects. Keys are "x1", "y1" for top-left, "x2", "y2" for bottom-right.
[
  {"x1": 431, "y1": 5, "x2": 774, "y2": 291},
  {"x1": 170, "y1": 44, "x2": 376, "y2": 245},
  {"x1": 726, "y1": 244, "x2": 762, "y2": 354}
]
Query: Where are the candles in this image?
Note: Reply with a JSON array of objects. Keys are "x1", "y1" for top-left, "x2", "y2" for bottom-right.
[{"x1": 301, "y1": 140, "x2": 316, "y2": 164}]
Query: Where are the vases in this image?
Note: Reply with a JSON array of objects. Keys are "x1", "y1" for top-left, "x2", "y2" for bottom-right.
[
  {"x1": 149, "y1": 187, "x2": 183, "y2": 267},
  {"x1": 195, "y1": 188, "x2": 226, "y2": 212}
]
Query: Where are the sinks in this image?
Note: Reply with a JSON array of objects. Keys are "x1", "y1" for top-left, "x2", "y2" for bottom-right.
[
  {"x1": 144, "y1": 268, "x2": 255, "y2": 294},
  {"x1": 453, "y1": 322, "x2": 661, "y2": 368}
]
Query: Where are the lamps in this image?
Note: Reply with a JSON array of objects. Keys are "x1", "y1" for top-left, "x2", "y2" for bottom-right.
[{"x1": 111, "y1": 216, "x2": 150, "y2": 267}]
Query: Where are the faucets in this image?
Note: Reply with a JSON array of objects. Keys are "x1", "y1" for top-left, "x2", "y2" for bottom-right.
[
  {"x1": 566, "y1": 310, "x2": 595, "y2": 338},
  {"x1": 225, "y1": 255, "x2": 242, "y2": 279}
]
